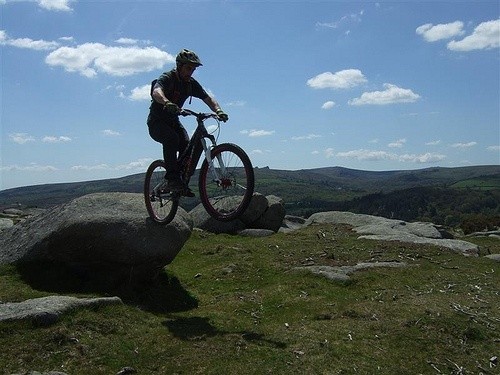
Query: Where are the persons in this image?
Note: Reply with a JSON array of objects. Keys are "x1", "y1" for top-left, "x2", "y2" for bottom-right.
[{"x1": 146, "y1": 49, "x2": 230, "y2": 197}]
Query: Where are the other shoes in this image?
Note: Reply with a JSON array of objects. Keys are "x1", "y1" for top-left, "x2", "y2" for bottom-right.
[{"x1": 166, "y1": 178, "x2": 195, "y2": 197}]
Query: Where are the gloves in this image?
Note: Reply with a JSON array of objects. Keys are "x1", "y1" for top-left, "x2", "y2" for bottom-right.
[
  {"x1": 165, "y1": 101, "x2": 179, "y2": 114},
  {"x1": 216, "y1": 110, "x2": 228, "y2": 122}
]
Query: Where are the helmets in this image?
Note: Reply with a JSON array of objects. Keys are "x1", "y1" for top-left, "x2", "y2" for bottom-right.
[{"x1": 176, "y1": 48, "x2": 203, "y2": 67}]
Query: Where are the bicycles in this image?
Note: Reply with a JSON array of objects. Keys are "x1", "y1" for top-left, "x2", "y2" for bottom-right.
[{"x1": 144, "y1": 109, "x2": 255, "y2": 225}]
